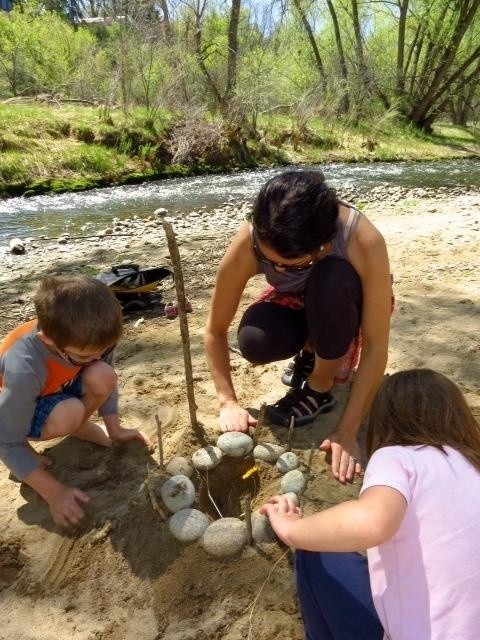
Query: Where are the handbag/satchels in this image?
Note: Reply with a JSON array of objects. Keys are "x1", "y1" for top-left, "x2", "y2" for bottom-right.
[{"x1": 95, "y1": 259, "x2": 176, "y2": 297}]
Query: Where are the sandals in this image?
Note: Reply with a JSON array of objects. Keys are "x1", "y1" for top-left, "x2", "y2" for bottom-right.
[{"x1": 262, "y1": 347, "x2": 338, "y2": 427}]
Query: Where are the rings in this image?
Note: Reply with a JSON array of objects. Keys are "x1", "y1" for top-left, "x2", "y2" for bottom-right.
[{"x1": 349, "y1": 456, "x2": 358, "y2": 461}]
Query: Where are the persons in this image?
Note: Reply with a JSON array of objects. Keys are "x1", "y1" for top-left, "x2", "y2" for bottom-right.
[
  {"x1": 0, "y1": 273, "x2": 144, "y2": 528},
  {"x1": 203, "y1": 169, "x2": 394, "y2": 485},
  {"x1": 259, "y1": 368, "x2": 480, "y2": 640}
]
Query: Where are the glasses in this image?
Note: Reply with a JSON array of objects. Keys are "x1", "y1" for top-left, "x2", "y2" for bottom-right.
[
  {"x1": 249, "y1": 228, "x2": 319, "y2": 273},
  {"x1": 44, "y1": 330, "x2": 118, "y2": 367}
]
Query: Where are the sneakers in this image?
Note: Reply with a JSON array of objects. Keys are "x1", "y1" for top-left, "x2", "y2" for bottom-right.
[
  {"x1": 116, "y1": 292, "x2": 165, "y2": 317},
  {"x1": 163, "y1": 297, "x2": 193, "y2": 318}
]
